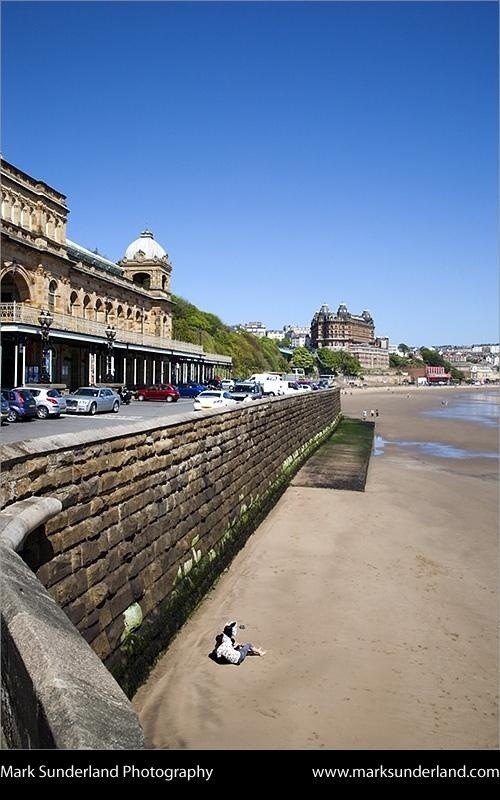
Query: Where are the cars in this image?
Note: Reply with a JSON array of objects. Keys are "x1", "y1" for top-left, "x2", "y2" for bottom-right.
[
  {"x1": 135, "y1": 383, "x2": 180, "y2": 403},
  {"x1": 245, "y1": 373, "x2": 331, "y2": 397},
  {"x1": 221, "y1": 379, "x2": 235, "y2": 390},
  {"x1": 175, "y1": 383, "x2": 205, "y2": 398},
  {"x1": 63, "y1": 387, "x2": 122, "y2": 415},
  {"x1": 207, "y1": 379, "x2": 223, "y2": 390},
  {"x1": 192, "y1": 389, "x2": 238, "y2": 413}
]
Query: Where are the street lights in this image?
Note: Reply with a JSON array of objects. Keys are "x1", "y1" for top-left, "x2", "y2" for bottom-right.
[
  {"x1": 36, "y1": 309, "x2": 54, "y2": 385},
  {"x1": 103, "y1": 326, "x2": 117, "y2": 382}
]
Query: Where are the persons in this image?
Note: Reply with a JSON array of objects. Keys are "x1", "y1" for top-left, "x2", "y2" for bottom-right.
[
  {"x1": 222, "y1": 620, "x2": 240, "y2": 649},
  {"x1": 214, "y1": 631, "x2": 266, "y2": 665},
  {"x1": 362, "y1": 407, "x2": 379, "y2": 420}
]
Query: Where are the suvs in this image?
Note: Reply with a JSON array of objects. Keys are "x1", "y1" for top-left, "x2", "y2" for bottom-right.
[
  {"x1": 223, "y1": 381, "x2": 264, "y2": 403},
  {"x1": 11, "y1": 387, "x2": 67, "y2": 420},
  {"x1": 1, "y1": 389, "x2": 36, "y2": 423},
  {"x1": 0, "y1": 393, "x2": 10, "y2": 424}
]
